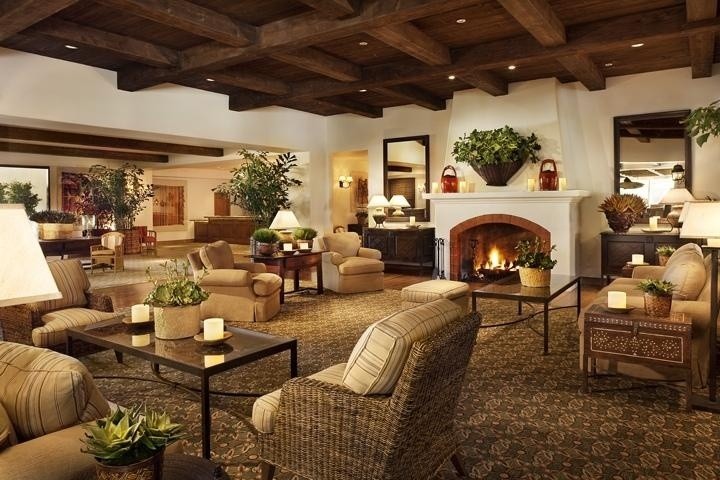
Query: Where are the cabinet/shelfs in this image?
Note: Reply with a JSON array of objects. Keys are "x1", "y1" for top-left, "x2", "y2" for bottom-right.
[{"x1": 190, "y1": 218, "x2": 255, "y2": 245}]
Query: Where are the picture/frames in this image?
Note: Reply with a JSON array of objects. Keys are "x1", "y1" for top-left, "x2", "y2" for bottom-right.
[{"x1": 0, "y1": 164, "x2": 50, "y2": 212}]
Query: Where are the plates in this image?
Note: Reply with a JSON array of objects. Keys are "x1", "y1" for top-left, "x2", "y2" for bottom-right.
[
  {"x1": 296, "y1": 248, "x2": 312, "y2": 253},
  {"x1": 599, "y1": 302, "x2": 636, "y2": 314},
  {"x1": 641, "y1": 229, "x2": 665, "y2": 233},
  {"x1": 281, "y1": 251, "x2": 296, "y2": 256},
  {"x1": 122, "y1": 315, "x2": 154, "y2": 324},
  {"x1": 627, "y1": 262, "x2": 649, "y2": 266},
  {"x1": 193, "y1": 331, "x2": 233, "y2": 345}
]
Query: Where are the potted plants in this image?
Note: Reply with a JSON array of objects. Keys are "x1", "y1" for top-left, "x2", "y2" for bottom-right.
[
  {"x1": 598, "y1": 194, "x2": 648, "y2": 233},
  {"x1": 212, "y1": 144, "x2": 303, "y2": 263},
  {"x1": 450, "y1": 125, "x2": 540, "y2": 187}
]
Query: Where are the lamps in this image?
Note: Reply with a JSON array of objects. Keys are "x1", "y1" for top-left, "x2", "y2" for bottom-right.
[
  {"x1": 0, "y1": 203, "x2": 64, "y2": 308},
  {"x1": 338, "y1": 176, "x2": 353, "y2": 188},
  {"x1": 619, "y1": 178, "x2": 643, "y2": 188},
  {"x1": 672, "y1": 165, "x2": 685, "y2": 180},
  {"x1": 389, "y1": 195, "x2": 410, "y2": 217},
  {"x1": 366, "y1": 195, "x2": 392, "y2": 228}
]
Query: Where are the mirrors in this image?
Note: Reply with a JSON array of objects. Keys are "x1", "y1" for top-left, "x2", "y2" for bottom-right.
[
  {"x1": 383, "y1": 134, "x2": 429, "y2": 222},
  {"x1": 613, "y1": 109, "x2": 692, "y2": 210}
]
